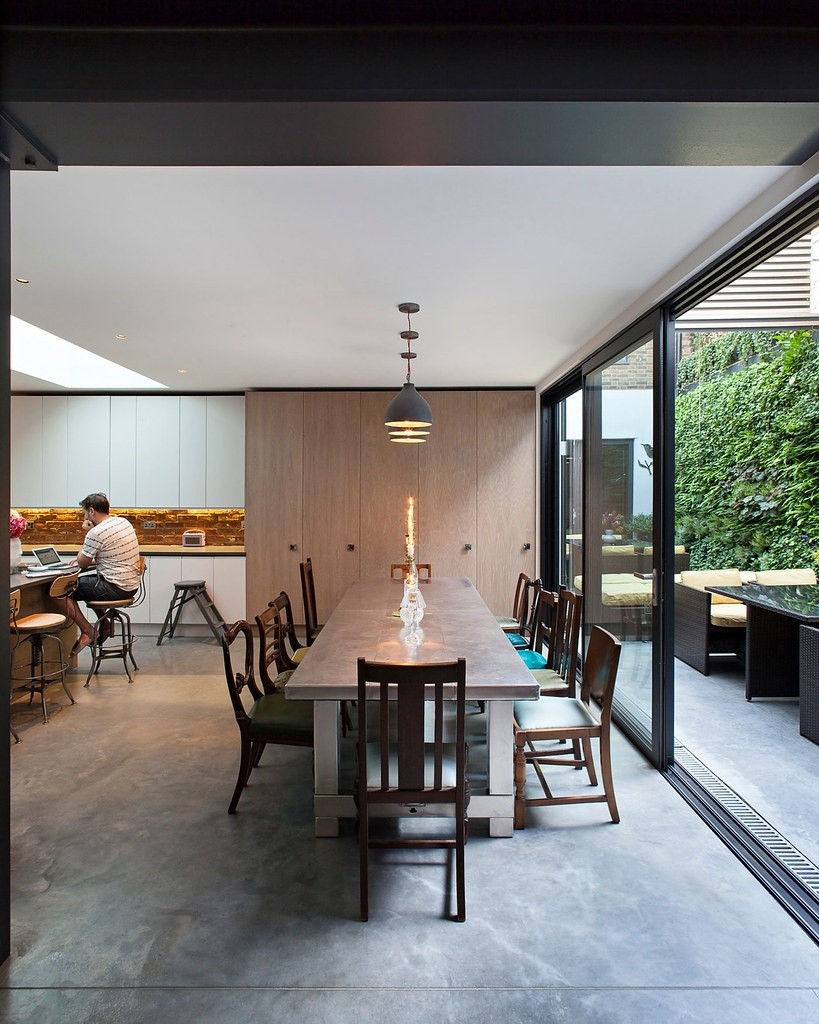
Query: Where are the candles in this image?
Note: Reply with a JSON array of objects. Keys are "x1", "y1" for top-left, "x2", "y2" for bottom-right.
[{"x1": 405, "y1": 497, "x2": 415, "y2": 560}]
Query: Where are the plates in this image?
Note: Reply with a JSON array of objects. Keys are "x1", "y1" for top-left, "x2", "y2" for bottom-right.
[{"x1": 27, "y1": 566, "x2": 48, "y2": 571}]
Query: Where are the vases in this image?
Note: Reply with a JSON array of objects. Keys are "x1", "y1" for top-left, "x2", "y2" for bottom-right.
[{"x1": 10, "y1": 538, "x2": 23, "y2": 575}]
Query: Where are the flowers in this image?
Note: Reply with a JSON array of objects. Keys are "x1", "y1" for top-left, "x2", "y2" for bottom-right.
[{"x1": 10, "y1": 512, "x2": 26, "y2": 539}]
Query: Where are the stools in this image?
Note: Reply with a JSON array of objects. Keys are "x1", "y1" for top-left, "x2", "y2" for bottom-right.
[{"x1": 10, "y1": 556, "x2": 147, "y2": 744}]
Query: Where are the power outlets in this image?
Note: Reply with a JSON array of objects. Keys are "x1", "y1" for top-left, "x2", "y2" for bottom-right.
[{"x1": 143, "y1": 522, "x2": 156, "y2": 530}]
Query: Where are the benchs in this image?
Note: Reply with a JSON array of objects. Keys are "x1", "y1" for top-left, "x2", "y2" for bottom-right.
[{"x1": 565, "y1": 534, "x2": 819, "y2": 747}]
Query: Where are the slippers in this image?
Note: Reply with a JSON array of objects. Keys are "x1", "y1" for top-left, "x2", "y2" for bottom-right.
[
  {"x1": 97, "y1": 622, "x2": 115, "y2": 644},
  {"x1": 68, "y1": 625, "x2": 100, "y2": 658}
]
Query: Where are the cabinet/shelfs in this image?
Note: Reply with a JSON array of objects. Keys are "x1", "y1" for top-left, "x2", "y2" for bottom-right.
[
  {"x1": 244, "y1": 387, "x2": 536, "y2": 638},
  {"x1": 19, "y1": 556, "x2": 246, "y2": 625},
  {"x1": 10, "y1": 395, "x2": 245, "y2": 509}
]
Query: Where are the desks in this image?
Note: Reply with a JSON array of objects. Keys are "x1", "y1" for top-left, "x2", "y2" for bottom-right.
[
  {"x1": 704, "y1": 584, "x2": 819, "y2": 702},
  {"x1": 10, "y1": 555, "x2": 96, "y2": 703},
  {"x1": 565, "y1": 539, "x2": 653, "y2": 549},
  {"x1": 284, "y1": 576, "x2": 540, "y2": 837}
]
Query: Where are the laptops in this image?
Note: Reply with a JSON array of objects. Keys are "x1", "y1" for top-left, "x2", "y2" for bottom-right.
[{"x1": 31, "y1": 546, "x2": 79, "y2": 570}]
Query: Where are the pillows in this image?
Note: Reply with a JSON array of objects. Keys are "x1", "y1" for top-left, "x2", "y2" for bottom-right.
[
  {"x1": 681, "y1": 570, "x2": 745, "y2": 603},
  {"x1": 645, "y1": 546, "x2": 685, "y2": 554},
  {"x1": 601, "y1": 545, "x2": 634, "y2": 555},
  {"x1": 753, "y1": 569, "x2": 817, "y2": 585}
]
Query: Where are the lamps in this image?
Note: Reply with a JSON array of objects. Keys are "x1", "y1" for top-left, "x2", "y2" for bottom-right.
[{"x1": 384, "y1": 303, "x2": 432, "y2": 443}]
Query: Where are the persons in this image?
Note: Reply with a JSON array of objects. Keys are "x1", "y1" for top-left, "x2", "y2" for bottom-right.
[{"x1": 49, "y1": 493, "x2": 142, "y2": 656}]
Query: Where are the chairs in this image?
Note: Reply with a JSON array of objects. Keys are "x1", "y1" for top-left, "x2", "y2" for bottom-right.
[{"x1": 222, "y1": 558, "x2": 623, "y2": 922}]
